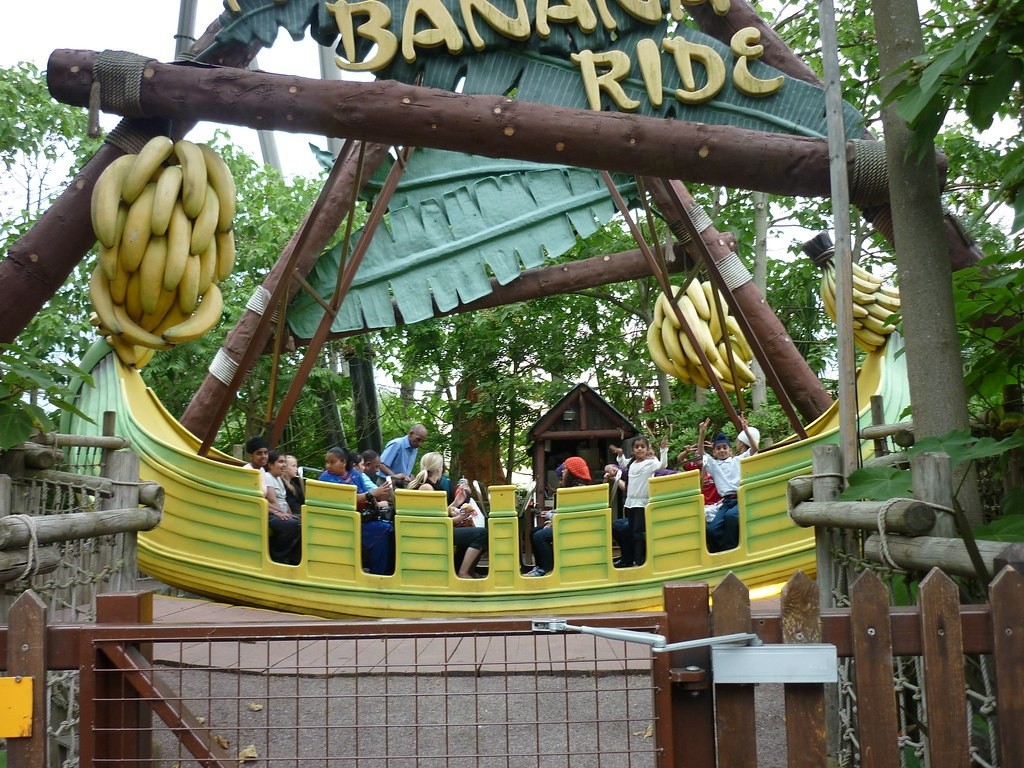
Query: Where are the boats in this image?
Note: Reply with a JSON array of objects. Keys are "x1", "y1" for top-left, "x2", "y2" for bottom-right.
[{"x1": 58, "y1": 318, "x2": 935, "y2": 618}]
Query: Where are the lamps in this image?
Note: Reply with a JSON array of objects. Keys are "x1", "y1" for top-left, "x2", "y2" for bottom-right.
[{"x1": 563, "y1": 408, "x2": 577, "y2": 421}]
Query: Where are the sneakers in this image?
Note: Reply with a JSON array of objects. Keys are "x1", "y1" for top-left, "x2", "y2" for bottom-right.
[{"x1": 522, "y1": 566, "x2": 545, "y2": 577}]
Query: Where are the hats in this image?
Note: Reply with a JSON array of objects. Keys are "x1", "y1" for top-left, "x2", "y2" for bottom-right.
[
  {"x1": 564, "y1": 457, "x2": 592, "y2": 480},
  {"x1": 737, "y1": 427, "x2": 760, "y2": 448},
  {"x1": 713, "y1": 431, "x2": 731, "y2": 447}
]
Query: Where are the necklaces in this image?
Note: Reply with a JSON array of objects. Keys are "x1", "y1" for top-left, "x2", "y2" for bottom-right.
[{"x1": 343, "y1": 476, "x2": 348, "y2": 480}]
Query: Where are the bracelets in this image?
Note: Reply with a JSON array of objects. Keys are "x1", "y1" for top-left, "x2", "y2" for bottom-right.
[
  {"x1": 272, "y1": 510, "x2": 279, "y2": 514},
  {"x1": 365, "y1": 492, "x2": 374, "y2": 501}
]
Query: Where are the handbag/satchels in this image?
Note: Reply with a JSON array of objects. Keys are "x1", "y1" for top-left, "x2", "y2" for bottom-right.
[{"x1": 360, "y1": 493, "x2": 394, "y2": 521}]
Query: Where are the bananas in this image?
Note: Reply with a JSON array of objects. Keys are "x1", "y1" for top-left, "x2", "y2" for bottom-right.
[
  {"x1": 819, "y1": 256, "x2": 903, "y2": 353},
  {"x1": 646, "y1": 277, "x2": 755, "y2": 393},
  {"x1": 90, "y1": 136, "x2": 234, "y2": 369}
]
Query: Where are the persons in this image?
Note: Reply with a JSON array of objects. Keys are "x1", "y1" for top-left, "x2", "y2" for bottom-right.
[
  {"x1": 519, "y1": 412, "x2": 765, "y2": 578},
  {"x1": 241, "y1": 414, "x2": 486, "y2": 579}
]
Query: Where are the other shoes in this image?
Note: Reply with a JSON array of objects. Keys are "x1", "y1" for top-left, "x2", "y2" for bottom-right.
[
  {"x1": 472, "y1": 574, "x2": 482, "y2": 577},
  {"x1": 615, "y1": 561, "x2": 633, "y2": 567}
]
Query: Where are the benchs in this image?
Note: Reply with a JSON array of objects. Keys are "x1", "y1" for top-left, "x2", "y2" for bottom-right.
[{"x1": 298, "y1": 454, "x2": 720, "y2": 519}]
